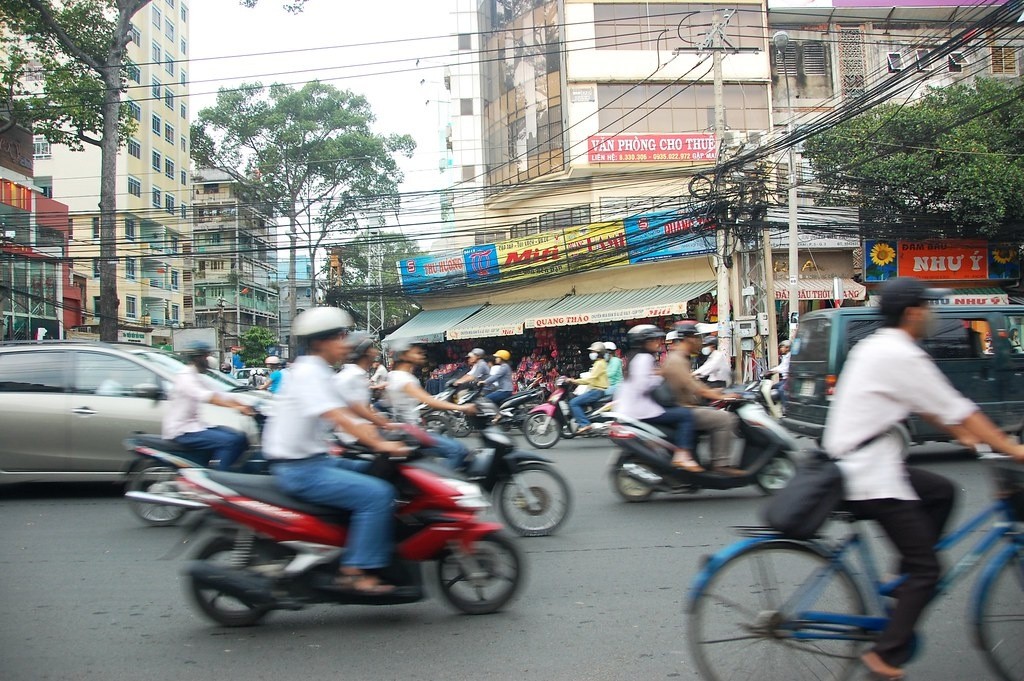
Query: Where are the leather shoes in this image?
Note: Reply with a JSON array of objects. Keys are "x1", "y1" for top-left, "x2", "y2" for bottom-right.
[
  {"x1": 672, "y1": 457, "x2": 706, "y2": 473},
  {"x1": 712, "y1": 464, "x2": 748, "y2": 474}
]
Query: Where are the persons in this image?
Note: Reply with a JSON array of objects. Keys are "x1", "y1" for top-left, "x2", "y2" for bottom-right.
[
  {"x1": 613, "y1": 322, "x2": 750, "y2": 476},
  {"x1": 91, "y1": 362, "x2": 127, "y2": 395},
  {"x1": 564, "y1": 341, "x2": 624, "y2": 435},
  {"x1": 162, "y1": 340, "x2": 266, "y2": 471},
  {"x1": 983, "y1": 338, "x2": 994, "y2": 355},
  {"x1": 259, "y1": 308, "x2": 477, "y2": 591},
  {"x1": 766, "y1": 338, "x2": 792, "y2": 415},
  {"x1": 1009, "y1": 328, "x2": 1023, "y2": 353},
  {"x1": 452, "y1": 348, "x2": 513, "y2": 426},
  {"x1": 822, "y1": 279, "x2": 1024, "y2": 681}
]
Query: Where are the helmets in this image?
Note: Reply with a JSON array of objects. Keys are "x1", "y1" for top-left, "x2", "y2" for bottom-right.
[
  {"x1": 265, "y1": 356, "x2": 279, "y2": 364},
  {"x1": 670, "y1": 319, "x2": 705, "y2": 337},
  {"x1": 602, "y1": 342, "x2": 618, "y2": 351},
  {"x1": 471, "y1": 348, "x2": 485, "y2": 358},
  {"x1": 586, "y1": 342, "x2": 606, "y2": 354},
  {"x1": 290, "y1": 306, "x2": 356, "y2": 336},
  {"x1": 701, "y1": 336, "x2": 719, "y2": 345},
  {"x1": 464, "y1": 352, "x2": 472, "y2": 358},
  {"x1": 222, "y1": 362, "x2": 231, "y2": 373},
  {"x1": 628, "y1": 324, "x2": 666, "y2": 350},
  {"x1": 493, "y1": 350, "x2": 511, "y2": 360},
  {"x1": 182, "y1": 340, "x2": 215, "y2": 356},
  {"x1": 779, "y1": 340, "x2": 792, "y2": 348},
  {"x1": 388, "y1": 340, "x2": 412, "y2": 361}
]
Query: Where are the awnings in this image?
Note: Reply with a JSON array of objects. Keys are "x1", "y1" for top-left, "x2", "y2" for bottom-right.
[
  {"x1": 1008, "y1": 296, "x2": 1024, "y2": 305},
  {"x1": 758, "y1": 277, "x2": 866, "y2": 300},
  {"x1": 379, "y1": 299, "x2": 485, "y2": 350},
  {"x1": 525, "y1": 279, "x2": 718, "y2": 328},
  {"x1": 446, "y1": 295, "x2": 565, "y2": 340},
  {"x1": 860, "y1": 286, "x2": 1009, "y2": 308}
]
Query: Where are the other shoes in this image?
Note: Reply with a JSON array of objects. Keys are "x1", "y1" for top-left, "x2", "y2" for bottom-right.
[
  {"x1": 575, "y1": 425, "x2": 592, "y2": 434},
  {"x1": 863, "y1": 652, "x2": 903, "y2": 680}
]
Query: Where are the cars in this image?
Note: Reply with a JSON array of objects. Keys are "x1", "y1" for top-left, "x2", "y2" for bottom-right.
[{"x1": 232, "y1": 368, "x2": 272, "y2": 386}]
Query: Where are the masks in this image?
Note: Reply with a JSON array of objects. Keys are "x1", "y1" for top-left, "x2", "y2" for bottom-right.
[
  {"x1": 701, "y1": 347, "x2": 712, "y2": 355},
  {"x1": 588, "y1": 352, "x2": 600, "y2": 361}
]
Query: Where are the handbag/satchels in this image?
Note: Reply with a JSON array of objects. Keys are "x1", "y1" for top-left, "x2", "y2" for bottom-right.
[{"x1": 768, "y1": 450, "x2": 843, "y2": 537}]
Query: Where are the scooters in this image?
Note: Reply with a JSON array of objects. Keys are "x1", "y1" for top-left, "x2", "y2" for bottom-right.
[
  {"x1": 523, "y1": 375, "x2": 620, "y2": 449},
  {"x1": 744, "y1": 370, "x2": 788, "y2": 404},
  {"x1": 126, "y1": 422, "x2": 522, "y2": 626},
  {"x1": 607, "y1": 371, "x2": 799, "y2": 503},
  {"x1": 414, "y1": 378, "x2": 544, "y2": 439},
  {"x1": 332, "y1": 402, "x2": 573, "y2": 537},
  {"x1": 124, "y1": 410, "x2": 267, "y2": 528},
  {"x1": 688, "y1": 374, "x2": 756, "y2": 412}
]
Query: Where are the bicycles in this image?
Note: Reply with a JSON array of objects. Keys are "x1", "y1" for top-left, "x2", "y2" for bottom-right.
[{"x1": 684, "y1": 452, "x2": 1024, "y2": 681}]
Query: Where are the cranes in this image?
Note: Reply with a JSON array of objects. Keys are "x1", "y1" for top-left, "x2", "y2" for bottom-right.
[
  {"x1": 325, "y1": 245, "x2": 351, "y2": 286},
  {"x1": 367, "y1": 229, "x2": 384, "y2": 345}
]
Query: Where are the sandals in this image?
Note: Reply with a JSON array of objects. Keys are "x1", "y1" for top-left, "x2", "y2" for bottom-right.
[{"x1": 331, "y1": 570, "x2": 394, "y2": 594}]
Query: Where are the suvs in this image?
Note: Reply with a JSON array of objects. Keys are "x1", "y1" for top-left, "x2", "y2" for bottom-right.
[{"x1": 0, "y1": 340, "x2": 272, "y2": 484}]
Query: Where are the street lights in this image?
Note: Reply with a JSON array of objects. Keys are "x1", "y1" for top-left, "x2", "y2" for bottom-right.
[{"x1": 772, "y1": 31, "x2": 800, "y2": 342}]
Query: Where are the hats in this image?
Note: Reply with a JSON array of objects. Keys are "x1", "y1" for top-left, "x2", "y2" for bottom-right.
[
  {"x1": 880, "y1": 278, "x2": 952, "y2": 299},
  {"x1": 346, "y1": 336, "x2": 373, "y2": 360}
]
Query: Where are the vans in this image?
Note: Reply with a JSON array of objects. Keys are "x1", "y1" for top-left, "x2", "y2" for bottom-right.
[{"x1": 779, "y1": 305, "x2": 1024, "y2": 453}]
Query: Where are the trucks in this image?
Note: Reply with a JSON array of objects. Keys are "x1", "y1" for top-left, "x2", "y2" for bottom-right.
[{"x1": 231, "y1": 345, "x2": 289, "y2": 376}]
